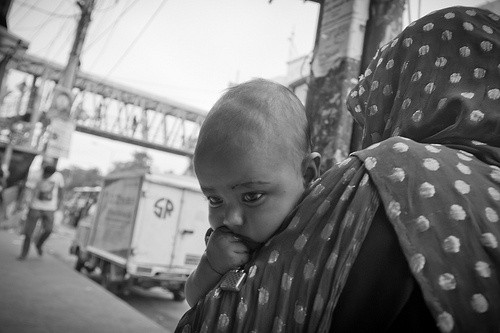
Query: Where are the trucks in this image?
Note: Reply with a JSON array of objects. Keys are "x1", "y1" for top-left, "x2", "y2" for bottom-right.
[{"x1": 69, "y1": 166, "x2": 211, "y2": 302}]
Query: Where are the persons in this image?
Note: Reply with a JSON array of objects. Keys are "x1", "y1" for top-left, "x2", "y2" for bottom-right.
[
  {"x1": 18, "y1": 165, "x2": 64, "y2": 260},
  {"x1": 175, "y1": 6, "x2": 500, "y2": 333},
  {"x1": 185, "y1": 78, "x2": 322, "y2": 309}
]
